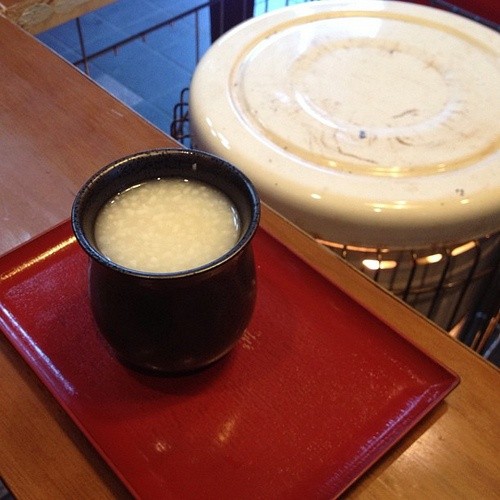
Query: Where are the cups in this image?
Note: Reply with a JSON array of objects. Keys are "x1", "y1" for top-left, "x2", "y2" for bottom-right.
[{"x1": 71, "y1": 147, "x2": 261, "y2": 374}]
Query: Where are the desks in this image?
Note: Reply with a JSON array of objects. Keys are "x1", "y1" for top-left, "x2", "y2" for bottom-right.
[{"x1": 0, "y1": 16, "x2": 500, "y2": 496}]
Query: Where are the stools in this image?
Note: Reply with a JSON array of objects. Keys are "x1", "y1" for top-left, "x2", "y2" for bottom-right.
[{"x1": 167, "y1": 0, "x2": 500, "y2": 342}]
